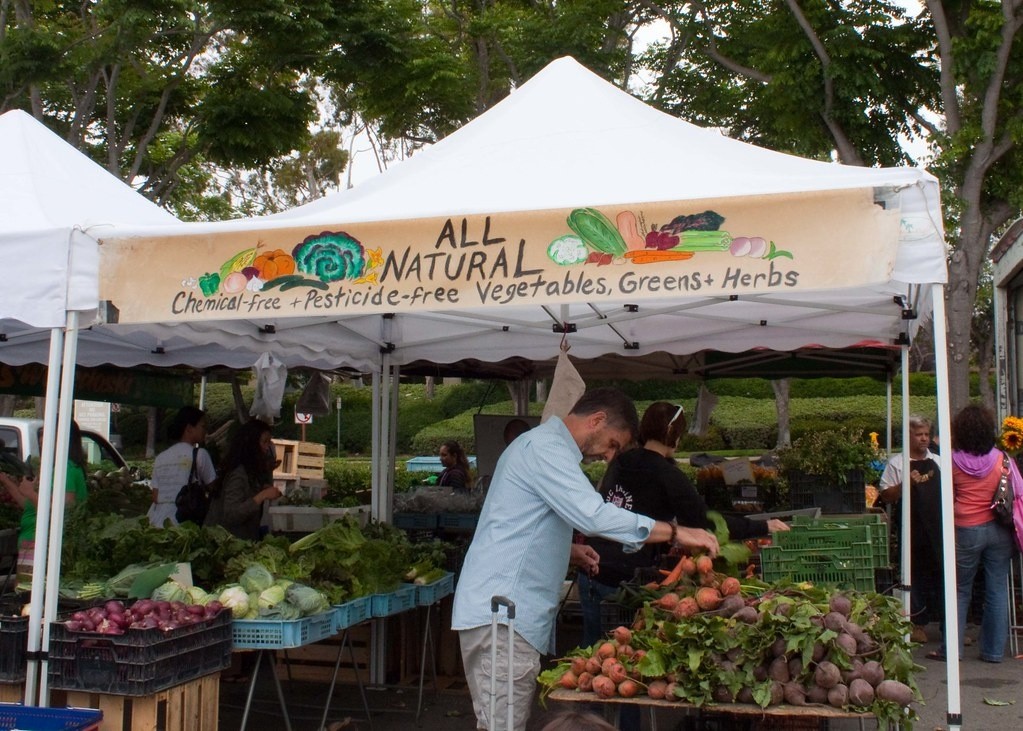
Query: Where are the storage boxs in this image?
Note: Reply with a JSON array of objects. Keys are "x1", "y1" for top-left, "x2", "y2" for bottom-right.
[
  {"x1": 0, "y1": 607, "x2": 237, "y2": 697},
  {"x1": 741, "y1": 467, "x2": 893, "y2": 594},
  {"x1": 267, "y1": 438, "x2": 373, "y2": 533}
]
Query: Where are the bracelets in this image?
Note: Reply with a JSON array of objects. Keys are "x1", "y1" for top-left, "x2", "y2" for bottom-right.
[{"x1": 667, "y1": 518, "x2": 681, "y2": 553}]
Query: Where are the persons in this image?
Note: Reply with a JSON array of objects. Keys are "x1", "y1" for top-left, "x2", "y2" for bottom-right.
[
  {"x1": 926, "y1": 402, "x2": 1014, "y2": 663},
  {"x1": 482, "y1": 419, "x2": 530, "y2": 497},
  {"x1": 201, "y1": 418, "x2": 282, "y2": 683},
  {"x1": 879, "y1": 414, "x2": 974, "y2": 645},
  {"x1": 448, "y1": 387, "x2": 721, "y2": 731},
  {"x1": 151, "y1": 405, "x2": 222, "y2": 533},
  {"x1": 577, "y1": 399, "x2": 791, "y2": 731},
  {"x1": 434, "y1": 438, "x2": 472, "y2": 494},
  {"x1": 538, "y1": 705, "x2": 620, "y2": 731},
  {"x1": 0, "y1": 415, "x2": 90, "y2": 613}
]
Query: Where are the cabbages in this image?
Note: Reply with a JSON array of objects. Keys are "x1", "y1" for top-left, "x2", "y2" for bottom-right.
[{"x1": 151, "y1": 564, "x2": 321, "y2": 642}]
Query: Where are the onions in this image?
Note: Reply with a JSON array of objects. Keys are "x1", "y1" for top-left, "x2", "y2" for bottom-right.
[{"x1": 64, "y1": 601, "x2": 222, "y2": 659}]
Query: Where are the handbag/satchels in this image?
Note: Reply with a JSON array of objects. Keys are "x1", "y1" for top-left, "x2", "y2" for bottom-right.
[
  {"x1": 990, "y1": 453, "x2": 1014, "y2": 532},
  {"x1": 174, "y1": 448, "x2": 211, "y2": 530}
]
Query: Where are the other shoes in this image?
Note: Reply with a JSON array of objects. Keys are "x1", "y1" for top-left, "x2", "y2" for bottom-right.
[
  {"x1": 911, "y1": 625, "x2": 928, "y2": 643},
  {"x1": 964, "y1": 637, "x2": 971, "y2": 645},
  {"x1": 925, "y1": 651, "x2": 946, "y2": 662}
]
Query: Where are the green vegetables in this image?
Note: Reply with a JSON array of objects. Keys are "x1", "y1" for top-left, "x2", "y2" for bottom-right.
[
  {"x1": 58, "y1": 496, "x2": 448, "y2": 605},
  {"x1": 757, "y1": 431, "x2": 881, "y2": 512}
]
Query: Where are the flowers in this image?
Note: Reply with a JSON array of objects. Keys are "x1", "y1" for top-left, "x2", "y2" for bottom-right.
[{"x1": 996, "y1": 415, "x2": 1023, "y2": 456}]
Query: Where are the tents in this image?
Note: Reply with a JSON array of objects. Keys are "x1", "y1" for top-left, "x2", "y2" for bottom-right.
[
  {"x1": 35, "y1": 54, "x2": 967, "y2": 731},
  {"x1": 0, "y1": 103, "x2": 394, "y2": 711}
]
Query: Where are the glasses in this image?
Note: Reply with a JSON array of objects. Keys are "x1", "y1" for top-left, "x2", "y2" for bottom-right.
[{"x1": 668, "y1": 405, "x2": 684, "y2": 427}]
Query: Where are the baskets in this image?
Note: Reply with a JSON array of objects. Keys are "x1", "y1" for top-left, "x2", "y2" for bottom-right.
[{"x1": 231, "y1": 568, "x2": 455, "y2": 649}]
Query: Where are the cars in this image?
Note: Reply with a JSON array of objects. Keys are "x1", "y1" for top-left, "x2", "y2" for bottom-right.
[{"x1": 0, "y1": 416, "x2": 152, "y2": 489}]
